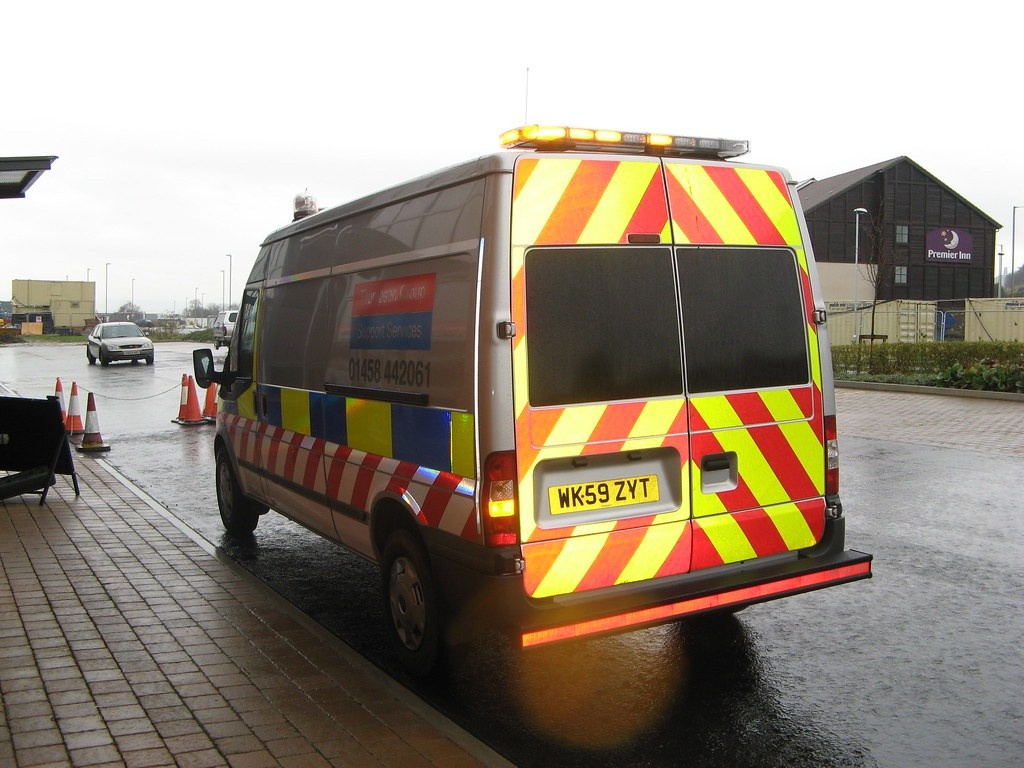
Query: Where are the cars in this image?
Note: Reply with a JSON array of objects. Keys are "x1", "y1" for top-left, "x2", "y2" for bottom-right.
[
  {"x1": 172, "y1": 324, "x2": 205, "y2": 336},
  {"x1": 86, "y1": 321, "x2": 154, "y2": 365}
]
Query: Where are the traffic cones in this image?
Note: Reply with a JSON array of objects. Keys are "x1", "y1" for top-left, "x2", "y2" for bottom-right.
[
  {"x1": 74, "y1": 391, "x2": 112, "y2": 453},
  {"x1": 64, "y1": 382, "x2": 86, "y2": 434},
  {"x1": 54, "y1": 376, "x2": 68, "y2": 425},
  {"x1": 202, "y1": 382, "x2": 217, "y2": 418},
  {"x1": 177, "y1": 375, "x2": 208, "y2": 426},
  {"x1": 171, "y1": 373, "x2": 188, "y2": 423},
  {"x1": 206, "y1": 381, "x2": 221, "y2": 422}
]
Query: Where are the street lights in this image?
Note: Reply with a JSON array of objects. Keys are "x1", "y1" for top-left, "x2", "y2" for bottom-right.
[
  {"x1": 194, "y1": 286, "x2": 200, "y2": 309},
  {"x1": 184, "y1": 296, "x2": 188, "y2": 314},
  {"x1": 220, "y1": 270, "x2": 227, "y2": 313},
  {"x1": 852, "y1": 207, "x2": 870, "y2": 345},
  {"x1": 1007, "y1": 205, "x2": 1023, "y2": 299},
  {"x1": 224, "y1": 254, "x2": 232, "y2": 310},
  {"x1": 86, "y1": 268, "x2": 92, "y2": 280},
  {"x1": 103, "y1": 262, "x2": 112, "y2": 314},
  {"x1": 200, "y1": 292, "x2": 206, "y2": 312},
  {"x1": 131, "y1": 277, "x2": 138, "y2": 310}
]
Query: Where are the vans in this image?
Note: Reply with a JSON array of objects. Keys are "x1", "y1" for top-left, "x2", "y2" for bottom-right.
[{"x1": 213, "y1": 310, "x2": 238, "y2": 349}]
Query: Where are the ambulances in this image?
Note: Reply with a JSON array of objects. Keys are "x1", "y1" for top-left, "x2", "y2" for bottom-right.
[{"x1": 193, "y1": 125, "x2": 875, "y2": 670}]
